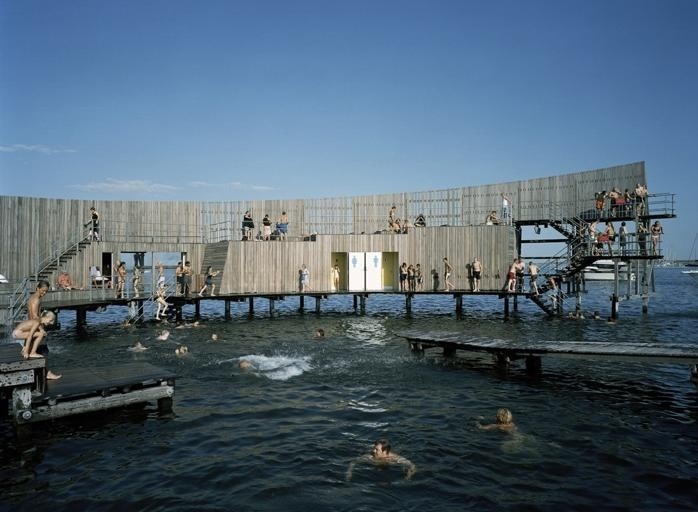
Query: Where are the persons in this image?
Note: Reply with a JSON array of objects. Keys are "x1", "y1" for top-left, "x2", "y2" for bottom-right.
[
  {"x1": 315, "y1": 328, "x2": 326, "y2": 337},
  {"x1": 301, "y1": 263, "x2": 313, "y2": 293},
  {"x1": 474, "y1": 407, "x2": 561, "y2": 458},
  {"x1": 262, "y1": 214, "x2": 272, "y2": 240},
  {"x1": 58, "y1": 269, "x2": 85, "y2": 292},
  {"x1": 84, "y1": 206, "x2": 101, "y2": 242},
  {"x1": 237, "y1": 360, "x2": 253, "y2": 368},
  {"x1": 344, "y1": 438, "x2": 418, "y2": 484},
  {"x1": 242, "y1": 211, "x2": 249, "y2": 239},
  {"x1": 115, "y1": 261, "x2": 221, "y2": 359},
  {"x1": 27, "y1": 279, "x2": 62, "y2": 380},
  {"x1": 332, "y1": 265, "x2": 341, "y2": 293},
  {"x1": 276, "y1": 212, "x2": 290, "y2": 242},
  {"x1": 247, "y1": 214, "x2": 255, "y2": 240},
  {"x1": 11, "y1": 309, "x2": 58, "y2": 359}
]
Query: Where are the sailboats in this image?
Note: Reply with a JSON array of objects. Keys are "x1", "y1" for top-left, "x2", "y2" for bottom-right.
[{"x1": 679, "y1": 232, "x2": 698, "y2": 285}]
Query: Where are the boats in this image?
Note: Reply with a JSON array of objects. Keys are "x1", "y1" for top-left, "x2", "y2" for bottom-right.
[{"x1": 554, "y1": 259, "x2": 638, "y2": 284}]
